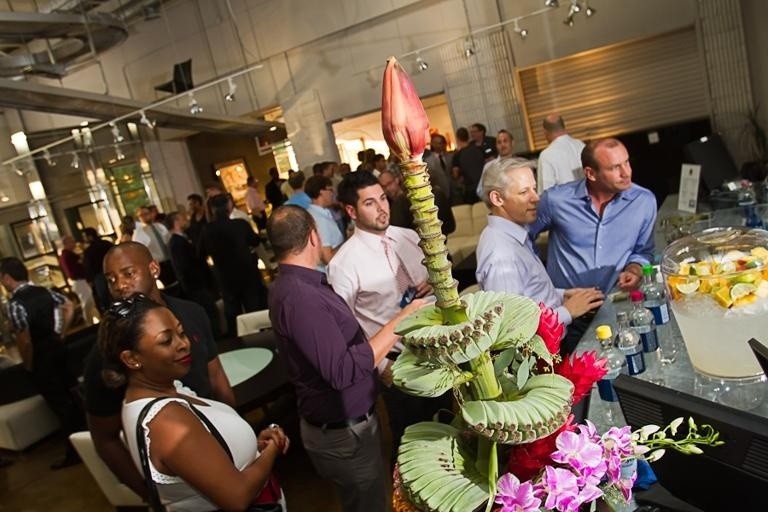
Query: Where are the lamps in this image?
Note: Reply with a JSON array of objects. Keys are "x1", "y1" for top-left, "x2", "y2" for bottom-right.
[
  {"x1": 0, "y1": 75, "x2": 235, "y2": 181},
  {"x1": 393, "y1": 1, "x2": 596, "y2": 74}
]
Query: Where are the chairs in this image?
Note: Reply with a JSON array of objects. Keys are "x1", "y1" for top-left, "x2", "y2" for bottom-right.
[
  {"x1": 67, "y1": 429, "x2": 154, "y2": 512},
  {"x1": 151, "y1": 57, "x2": 194, "y2": 109}
]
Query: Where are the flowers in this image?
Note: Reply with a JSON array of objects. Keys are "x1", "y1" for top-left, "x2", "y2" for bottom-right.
[{"x1": 380, "y1": 55, "x2": 724, "y2": 512}]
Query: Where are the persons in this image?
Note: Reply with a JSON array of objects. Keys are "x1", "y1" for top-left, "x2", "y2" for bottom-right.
[
  {"x1": 537, "y1": 114, "x2": 587, "y2": 242},
  {"x1": 474, "y1": 153, "x2": 604, "y2": 370},
  {"x1": 528, "y1": 138, "x2": 659, "y2": 430}
]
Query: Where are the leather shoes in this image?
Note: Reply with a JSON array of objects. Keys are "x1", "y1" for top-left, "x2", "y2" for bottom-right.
[{"x1": 50, "y1": 457, "x2": 81, "y2": 470}]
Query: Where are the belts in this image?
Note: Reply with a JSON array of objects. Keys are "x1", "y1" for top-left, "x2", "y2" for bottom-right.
[{"x1": 300, "y1": 402, "x2": 375, "y2": 431}]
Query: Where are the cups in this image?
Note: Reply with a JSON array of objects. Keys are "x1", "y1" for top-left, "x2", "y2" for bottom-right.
[{"x1": 660, "y1": 226, "x2": 768, "y2": 382}]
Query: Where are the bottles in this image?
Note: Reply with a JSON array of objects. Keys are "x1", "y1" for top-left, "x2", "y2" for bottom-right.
[
  {"x1": 737, "y1": 181, "x2": 762, "y2": 228},
  {"x1": 592, "y1": 324, "x2": 628, "y2": 426},
  {"x1": 627, "y1": 289, "x2": 668, "y2": 384},
  {"x1": 613, "y1": 311, "x2": 649, "y2": 381},
  {"x1": 638, "y1": 265, "x2": 679, "y2": 363}
]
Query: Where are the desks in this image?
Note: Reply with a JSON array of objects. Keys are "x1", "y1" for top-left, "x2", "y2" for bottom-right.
[{"x1": 214, "y1": 346, "x2": 275, "y2": 389}]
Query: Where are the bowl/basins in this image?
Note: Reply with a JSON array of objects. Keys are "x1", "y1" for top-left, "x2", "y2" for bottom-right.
[{"x1": 660, "y1": 214, "x2": 716, "y2": 246}]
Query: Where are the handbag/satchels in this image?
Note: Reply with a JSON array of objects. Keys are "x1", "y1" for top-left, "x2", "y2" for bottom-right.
[{"x1": 135, "y1": 398, "x2": 285, "y2": 512}]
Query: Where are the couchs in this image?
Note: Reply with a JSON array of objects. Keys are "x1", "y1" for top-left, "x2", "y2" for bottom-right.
[{"x1": 445, "y1": 200, "x2": 553, "y2": 268}]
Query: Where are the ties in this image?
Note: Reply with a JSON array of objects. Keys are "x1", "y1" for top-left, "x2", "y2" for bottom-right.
[
  {"x1": 381, "y1": 238, "x2": 416, "y2": 297},
  {"x1": 149, "y1": 223, "x2": 174, "y2": 262},
  {"x1": 439, "y1": 154, "x2": 446, "y2": 172}
]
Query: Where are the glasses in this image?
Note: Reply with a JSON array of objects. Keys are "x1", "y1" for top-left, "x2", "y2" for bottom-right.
[{"x1": 108, "y1": 293, "x2": 149, "y2": 337}]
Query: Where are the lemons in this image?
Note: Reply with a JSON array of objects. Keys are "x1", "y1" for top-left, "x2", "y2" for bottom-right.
[{"x1": 731, "y1": 281, "x2": 756, "y2": 299}]
